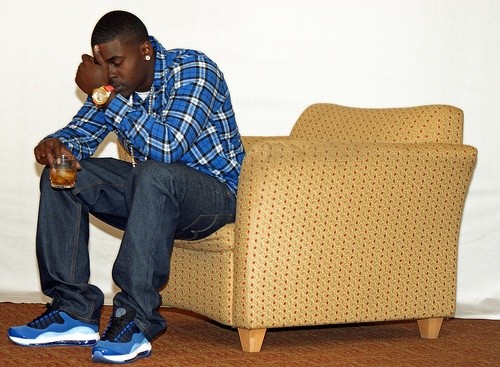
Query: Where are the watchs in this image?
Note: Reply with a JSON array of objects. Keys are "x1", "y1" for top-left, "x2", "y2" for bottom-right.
[{"x1": 91, "y1": 85, "x2": 116, "y2": 108}]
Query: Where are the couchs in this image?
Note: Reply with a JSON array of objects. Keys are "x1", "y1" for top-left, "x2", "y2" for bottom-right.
[{"x1": 115, "y1": 104, "x2": 479, "y2": 354}]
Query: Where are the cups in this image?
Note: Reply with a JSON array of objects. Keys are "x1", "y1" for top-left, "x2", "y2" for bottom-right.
[{"x1": 50, "y1": 155, "x2": 76, "y2": 189}]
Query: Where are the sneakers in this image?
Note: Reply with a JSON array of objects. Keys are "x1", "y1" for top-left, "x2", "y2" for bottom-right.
[
  {"x1": 8, "y1": 301, "x2": 100, "y2": 346},
  {"x1": 91, "y1": 303, "x2": 151, "y2": 365}
]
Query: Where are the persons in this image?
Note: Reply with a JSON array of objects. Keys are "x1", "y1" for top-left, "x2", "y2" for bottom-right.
[{"x1": 7, "y1": 10, "x2": 245, "y2": 364}]
